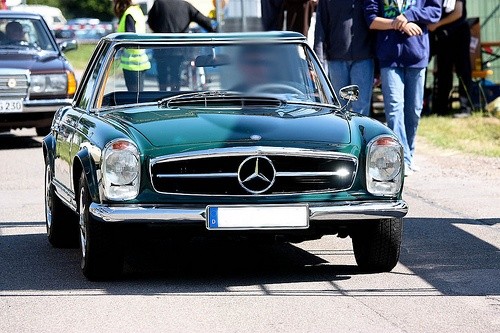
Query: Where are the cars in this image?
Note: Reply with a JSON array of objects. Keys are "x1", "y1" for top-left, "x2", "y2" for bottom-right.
[
  {"x1": 41, "y1": 28, "x2": 407, "y2": 280},
  {"x1": 0, "y1": 10, "x2": 80, "y2": 138}
]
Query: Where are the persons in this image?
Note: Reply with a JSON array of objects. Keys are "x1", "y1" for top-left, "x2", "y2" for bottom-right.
[
  {"x1": 113, "y1": 0, "x2": 228, "y2": 91},
  {"x1": 0, "y1": 22, "x2": 29, "y2": 45},
  {"x1": 226, "y1": 43, "x2": 322, "y2": 102},
  {"x1": 260, "y1": 0, "x2": 492, "y2": 176}
]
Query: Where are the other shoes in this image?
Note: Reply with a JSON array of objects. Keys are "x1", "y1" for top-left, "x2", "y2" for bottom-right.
[
  {"x1": 453, "y1": 112, "x2": 469, "y2": 118},
  {"x1": 409, "y1": 161, "x2": 417, "y2": 171},
  {"x1": 404, "y1": 163, "x2": 410, "y2": 176}
]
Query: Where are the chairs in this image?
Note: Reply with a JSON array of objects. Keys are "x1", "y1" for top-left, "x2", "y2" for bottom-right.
[{"x1": 446, "y1": 48, "x2": 500, "y2": 119}]
